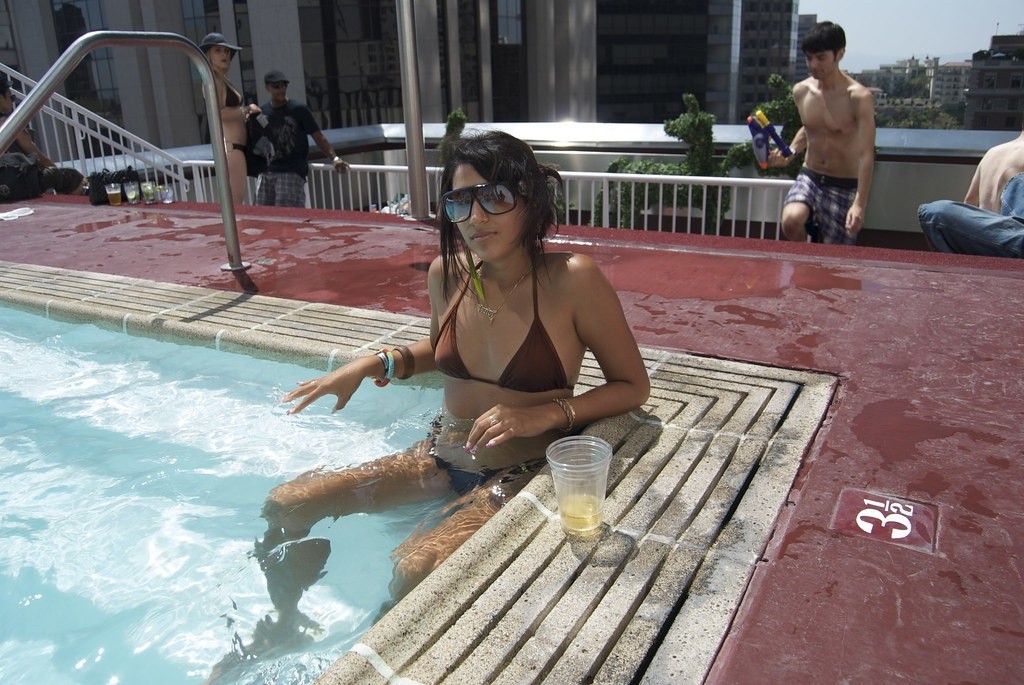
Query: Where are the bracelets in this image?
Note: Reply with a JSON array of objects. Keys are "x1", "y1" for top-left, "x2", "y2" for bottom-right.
[
  {"x1": 373, "y1": 344, "x2": 416, "y2": 387},
  {"x1": 241, "y1": 107, "x2": 244, "y2": 116},
  {"x1": 245, "y1": 106, "x2": 253, "y2": 115},
  {"x1": 551, "y1": 397, "x2": 576, "y2": 432}
]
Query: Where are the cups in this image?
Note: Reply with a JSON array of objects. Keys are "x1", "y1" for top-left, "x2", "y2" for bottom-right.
[
  {"x1": 124, "y1": 182, "x2": 140, "y2": 204},
  {"x1": 104, "y1": 183, "x2": 121, "y2": 205},
  {"x1": 158, "y1": 185, "x2": 174, "y2": 203},
  {"x1": 141, "y1": 182, "x2": 156, "y2": 205},
  {"x1": 545, "y1": 436, "x2": 613, "y2": 532}
]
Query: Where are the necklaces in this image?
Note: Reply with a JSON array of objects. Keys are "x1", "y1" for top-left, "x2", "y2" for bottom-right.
[{"x1": 475, "y1": 260, "x2": 535, "y2": 325}]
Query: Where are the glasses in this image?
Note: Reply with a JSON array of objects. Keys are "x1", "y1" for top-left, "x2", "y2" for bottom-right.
[
  {"x1": 442, "y1": 182, "x2": 518, "y2": 223},
  {"x1": 270, "y1": 82, "x2": 287, "y2": 89},
  {"x1": 5, "y1": 94, "x2": 16, "y2": 101}
]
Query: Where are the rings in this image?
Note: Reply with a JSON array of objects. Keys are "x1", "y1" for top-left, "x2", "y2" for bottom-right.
[
  {"x1": 509, "y1": 427, "x2": 517, "y2": 438},
  {"x1": 311, "y1": 381, "x2": 316, "y2": 388},
  {"x1": 490, "y1": 416, "x2": 499, "y2": 426}
]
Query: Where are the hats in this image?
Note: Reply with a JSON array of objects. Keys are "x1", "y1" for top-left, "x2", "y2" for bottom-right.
[
  {"x1": 264, "y1": 71, "x2": 290, "y2": 84},
  {"x1": 0, "y1": 71, "x2": 13, "y2": 88},
  {"x1": 200, "y1": 33, "x2": 242, "y2": 61}
]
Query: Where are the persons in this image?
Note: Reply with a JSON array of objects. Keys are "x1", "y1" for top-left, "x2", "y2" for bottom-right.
[
  {"x1": 766, "y1": 20, "x2": 876, "y2": 247},
  {"x1": 290, "y1": 130, "x2": 652, "y2": 454},
  {"x1": 247, "y1": 96, "x2": 254, "y2": 105},
  {"x1": 198, "y1": 33, "x2": 263, "y2": 205},
  {"x1": 0, "y1": 75, "x2": 88, "y2": 203},
  {"x1": 917, "y1": 116, "x2": 1024, "y2": 261},
  {"x1": 245, "y1": 71, "x2": 351, "y2": 208}
]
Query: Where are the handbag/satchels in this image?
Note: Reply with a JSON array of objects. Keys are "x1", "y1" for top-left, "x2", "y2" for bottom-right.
[
  {"x1": 0, "y1": 151, "x2": 42, "y2": 202},
  {"x1": 246, "y1": 111, "x2": 277, "y2": 178},
  {"x1": 87, "y1": 165, "x2": 143, "y2": 205}
]
